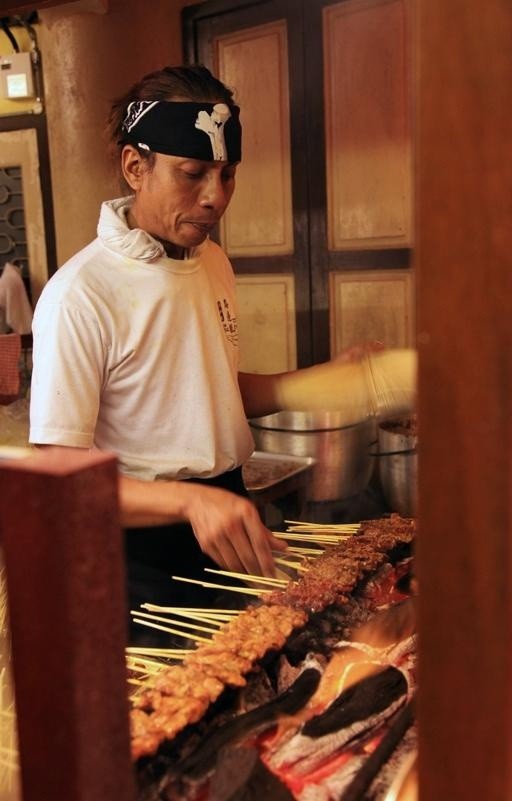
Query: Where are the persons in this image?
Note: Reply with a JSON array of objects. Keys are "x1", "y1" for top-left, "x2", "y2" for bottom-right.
[{"x1": 25, "y1": 58, "x2": 395, "y2": 664}]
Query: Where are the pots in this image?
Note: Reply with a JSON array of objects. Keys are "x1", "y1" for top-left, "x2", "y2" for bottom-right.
[
  {"x1": 379, "y1": 420, "x2": 418, "y2": 519},
  {"x1": 248, "y1": 410, "x2": 380, "y2": 502}
]
